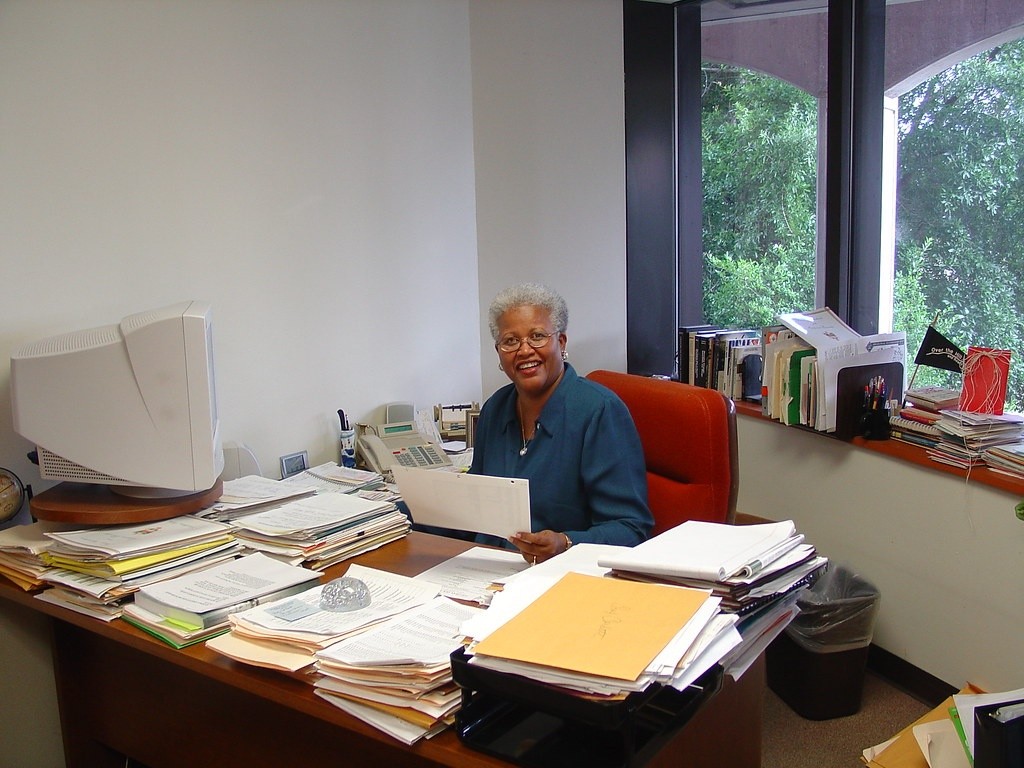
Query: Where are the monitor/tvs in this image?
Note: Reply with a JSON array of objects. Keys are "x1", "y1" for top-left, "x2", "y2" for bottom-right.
[{"x1": 11, "y1": 300, "x2": 224, "y2": 499}]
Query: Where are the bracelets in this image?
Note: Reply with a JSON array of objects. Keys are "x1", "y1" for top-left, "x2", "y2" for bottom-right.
[{"x1": 560, "y1": 532, "x2": 573, "y2": 550}]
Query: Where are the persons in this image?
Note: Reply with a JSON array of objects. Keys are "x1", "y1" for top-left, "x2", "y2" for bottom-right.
[{"x1": 391, "y1": 279, "x2": 656, "y2": 566}]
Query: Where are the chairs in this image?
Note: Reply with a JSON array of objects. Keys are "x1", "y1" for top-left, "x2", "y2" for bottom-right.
[{"x1": 586, "y1": 369, "x2": 739, "y2": 543}]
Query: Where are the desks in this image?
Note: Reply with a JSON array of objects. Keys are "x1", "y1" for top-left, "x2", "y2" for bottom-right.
[{"x1": 0, "y1": 528, "x2": 764, "y2": 768}]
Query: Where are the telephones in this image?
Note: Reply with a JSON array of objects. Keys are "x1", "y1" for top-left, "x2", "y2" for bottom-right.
[{"x1": 356, "y1": 421, "x2": 453, "y2": 477}]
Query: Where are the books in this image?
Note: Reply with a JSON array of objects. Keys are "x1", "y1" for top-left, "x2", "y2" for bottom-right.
[
  {"x1": 121, "y1": 458, "x2": 831, "y2": 746},
  {"x1": 677, "y1": 305, "x2": 1024, "y2": 481}
]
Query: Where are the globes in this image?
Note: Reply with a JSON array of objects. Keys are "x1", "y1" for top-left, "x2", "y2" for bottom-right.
[{"x1": 0, "y1": 467, "x2": 38, "y2": 524}]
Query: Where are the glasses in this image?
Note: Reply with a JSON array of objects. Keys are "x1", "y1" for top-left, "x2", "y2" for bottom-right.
[{"x1": 496, "y1": 329, "x2": 559, "y2": 352}]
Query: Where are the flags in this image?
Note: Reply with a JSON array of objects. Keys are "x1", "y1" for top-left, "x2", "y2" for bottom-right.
[{"x1": 914, "y1": 324, "x2": 967, "y2": 373}]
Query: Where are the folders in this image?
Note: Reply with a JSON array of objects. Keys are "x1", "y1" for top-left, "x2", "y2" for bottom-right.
[{"x1": 760, "y1": 325, "x2": 788, "y2": 416}]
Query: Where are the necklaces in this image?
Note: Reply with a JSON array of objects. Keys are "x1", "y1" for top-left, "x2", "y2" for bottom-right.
[{"x1": 518, "y1": 394, "x2": 535, "y2": 456}]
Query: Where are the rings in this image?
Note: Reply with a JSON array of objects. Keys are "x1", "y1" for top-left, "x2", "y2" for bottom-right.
[{"x1": 530, "y1": 555, "x2": 536, "y2": 566}]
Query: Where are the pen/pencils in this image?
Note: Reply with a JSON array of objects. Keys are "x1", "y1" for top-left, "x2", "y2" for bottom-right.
[
  {"x1": 345, "y1": 414, "x2": 353, "y2": 430},
  {"x1": 865, "y1": 376, "x2": 887, "y2": 409}
]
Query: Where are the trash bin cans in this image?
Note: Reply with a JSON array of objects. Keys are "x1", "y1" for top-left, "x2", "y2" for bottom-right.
[{"x1": 763, "y1": 564, "x2": 880, "y2": 720}]
